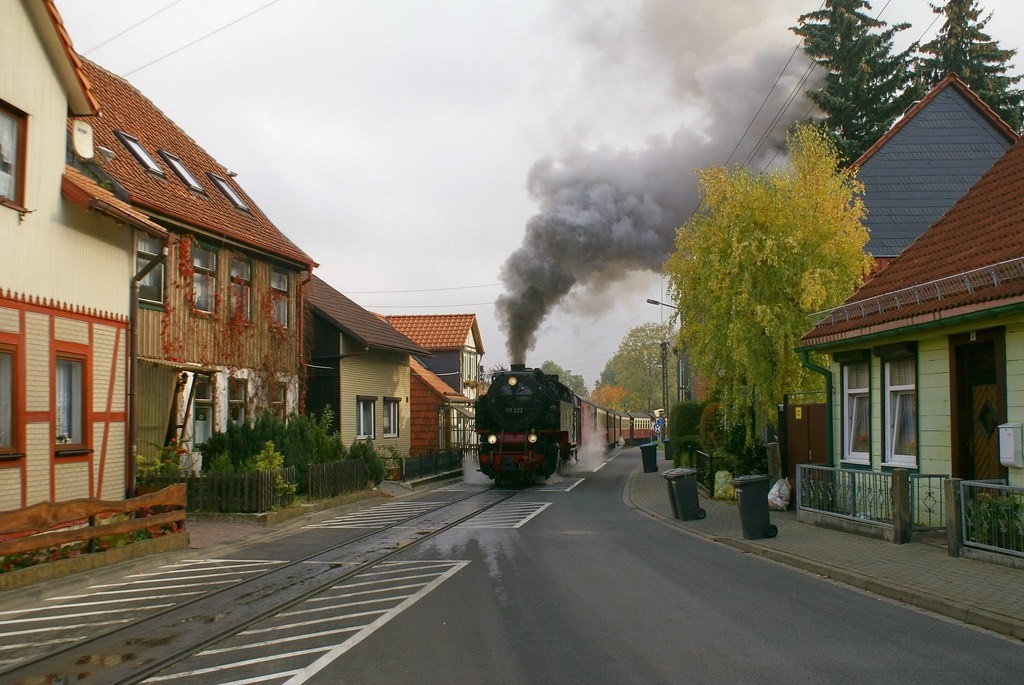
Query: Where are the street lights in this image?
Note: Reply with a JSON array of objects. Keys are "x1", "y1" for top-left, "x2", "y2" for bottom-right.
[{"x1": 647, "y1": 298, "x2": 689, "y2": 400}]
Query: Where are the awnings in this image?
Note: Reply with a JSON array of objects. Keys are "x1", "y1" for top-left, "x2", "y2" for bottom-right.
[{"x1": 453, "y1": 405, "x2": 475, "y2": 419}]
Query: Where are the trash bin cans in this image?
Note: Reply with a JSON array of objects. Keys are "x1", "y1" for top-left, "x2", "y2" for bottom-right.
[
  {"x1": 728, "y1": 473, "x2": 779, "y2": 539},
  {"x1": 639, "y1": 443, "x2": 659, "y2": 472},
  {"x1": 657, "y1": 467, "x2": 705, "y2": 520},
  {"x1": 664, "y1": 439, "x2": 672, "y2": 461}
]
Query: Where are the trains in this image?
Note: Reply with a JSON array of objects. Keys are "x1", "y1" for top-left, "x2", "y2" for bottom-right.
[{"x1": 474, "y1": 362, "x2": 665, "y2": 488}]
